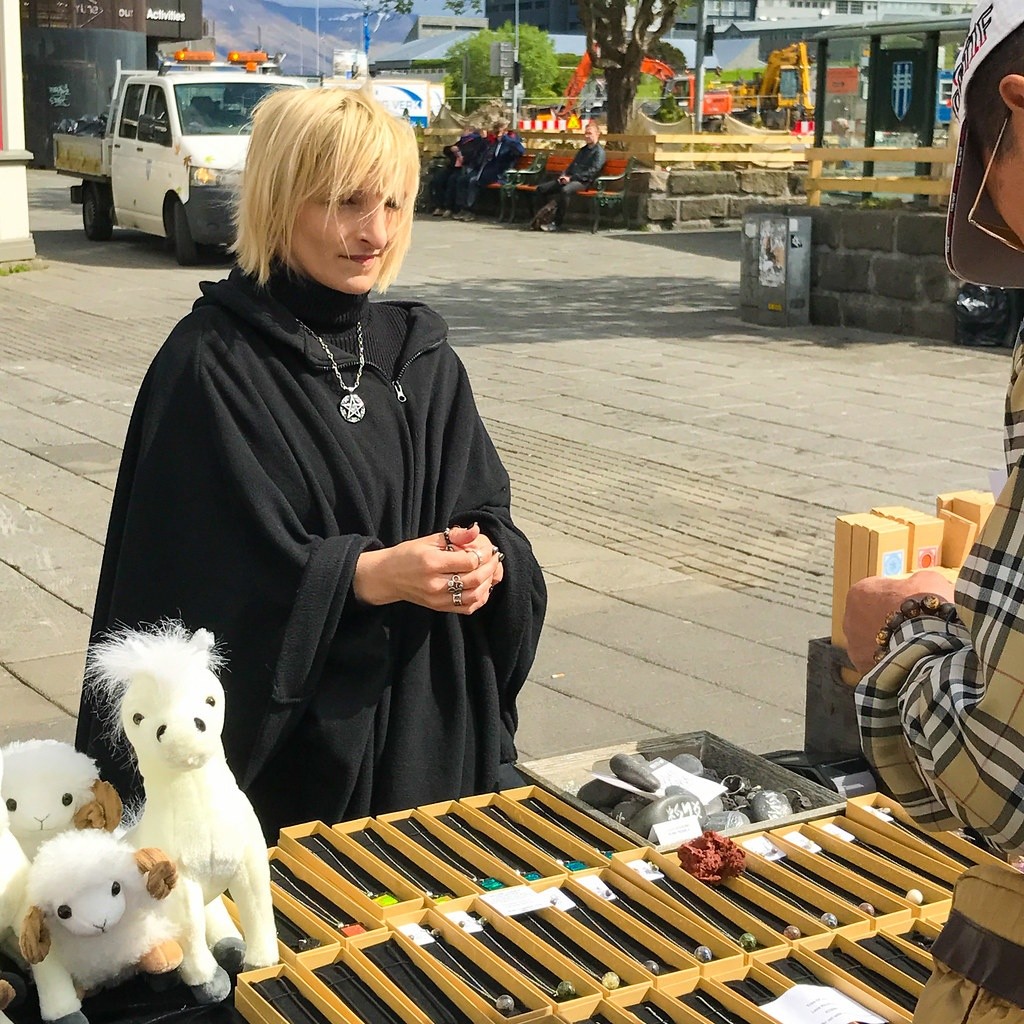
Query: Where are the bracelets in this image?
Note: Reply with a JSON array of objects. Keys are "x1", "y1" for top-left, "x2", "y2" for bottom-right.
[{"x1": 872, "y1": 594, "x2": 965, "y2": 667}]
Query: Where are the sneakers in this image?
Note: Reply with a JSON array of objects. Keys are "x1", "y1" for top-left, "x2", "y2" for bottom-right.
[{"x1": 540, "y1": 221, "x2": 560, "y2": 232}]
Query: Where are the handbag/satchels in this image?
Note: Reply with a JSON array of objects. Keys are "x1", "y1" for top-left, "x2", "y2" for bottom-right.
[{"x1": 528, "y1": 198, "x2": 559, "y2": 230}]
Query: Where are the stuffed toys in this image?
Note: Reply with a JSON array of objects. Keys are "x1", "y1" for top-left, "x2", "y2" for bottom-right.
[
  {"x1": 0, "y1": 750, "x2": 32, "y2": 928},
  {"x1": 0, "y1": 829, "x2": 185, "y2": 1024},
  {"x1": 84, "y1": 616, "x2": 280, "y2": 1002},
  {"x1": 0, "y1": 739, "x2": 123, "y2": 865},
  {"x1": 0, "y1": 979, "x2": 16, "y2": 1024}
]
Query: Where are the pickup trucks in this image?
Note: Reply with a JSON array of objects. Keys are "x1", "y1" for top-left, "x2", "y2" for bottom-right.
[{"x1": 50, "y1": 46, "x2": 310, "y2": 263}]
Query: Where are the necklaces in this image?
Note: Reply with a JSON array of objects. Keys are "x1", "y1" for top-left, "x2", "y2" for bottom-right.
[{"x1": 295, "y1": 316, "x2": 366, "y2": 423}]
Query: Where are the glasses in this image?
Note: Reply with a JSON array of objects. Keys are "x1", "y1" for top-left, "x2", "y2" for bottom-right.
[{"x1": 967, "y1": 108, "x2": 1024, "y2": 255}]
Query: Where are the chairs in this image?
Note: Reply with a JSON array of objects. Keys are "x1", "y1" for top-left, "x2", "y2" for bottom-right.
[{"x1": 182, "y1": 96, "x2": 227, "y2": 126}]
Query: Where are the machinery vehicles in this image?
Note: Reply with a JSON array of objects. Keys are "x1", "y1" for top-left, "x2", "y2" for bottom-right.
[
  {"x1": 708, "y1": 71, "x2": 765, "y2": 119},
  {"x1": 552, "y1": 38, "x2": 734, "y2": 132},
  {"x1": 756, "y1": 42, "x2": 815, "y2": 131}
]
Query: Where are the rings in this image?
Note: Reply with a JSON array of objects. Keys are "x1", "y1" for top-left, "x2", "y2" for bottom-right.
[
  {"x1": 452, "y1": 593, "x2": 462, "y2": 605},
  {"x1": 444, "y1": 528, "x2": 453, "y2": 545},
  {"x1": 447, "y1": 573, "x2": 463, "y2": 593},
  {"x1": 473, "y1": 550, "x2": 482, "y2": 566}
]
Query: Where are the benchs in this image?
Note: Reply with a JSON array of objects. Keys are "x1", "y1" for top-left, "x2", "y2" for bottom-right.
[
  {"x1": 484, "y1": 152, "x2": 542, "y2": 224},
  {"x1": 504, "y1": 151, "x2": 639, "y2": 235}
]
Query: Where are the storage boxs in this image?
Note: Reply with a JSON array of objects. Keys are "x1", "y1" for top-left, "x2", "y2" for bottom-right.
[{"x1": 234, "y1": 488, "x2": 1024, "y2": 1024}]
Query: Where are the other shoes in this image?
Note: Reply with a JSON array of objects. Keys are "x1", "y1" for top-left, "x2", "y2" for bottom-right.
[
  {"x1": 452, "y1": 210, "x2": 477, "y2": 222},
  {"x1": 442, "y1": 209, "x2": 452, "y2": 218},
  {"x1": 433, "y1": 208, "x2": 444, "y2": 215}
]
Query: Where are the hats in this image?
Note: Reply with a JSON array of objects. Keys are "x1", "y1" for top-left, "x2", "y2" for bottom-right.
[{"x1": 944, "y1": 0, "x2": 1024, "y2": 290}]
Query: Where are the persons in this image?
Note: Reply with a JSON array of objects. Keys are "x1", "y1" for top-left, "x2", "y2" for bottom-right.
[
  {"x1": 842, "y1": 0, "x2": 1024, "y2": 1024},
  {"x1": 75, "y1": 86, "x2": 548, "y2": 847},
  {"x1": 532, "y1": 123, "x2": 607, "y2": 232},
  {"x1": 426, "y1": 117, "x2": 525, "y2": 222}
]
